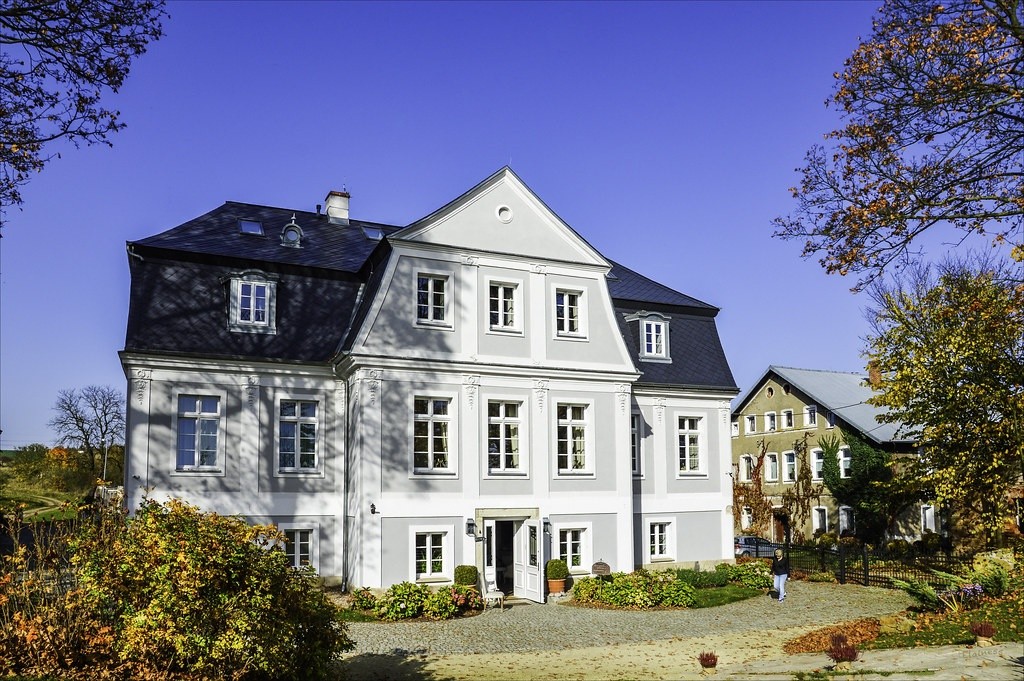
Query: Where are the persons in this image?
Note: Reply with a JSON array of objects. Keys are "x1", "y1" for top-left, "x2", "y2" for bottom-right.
[{"x1": 770, "y1": 550, "x2": 790, "y2": 602}]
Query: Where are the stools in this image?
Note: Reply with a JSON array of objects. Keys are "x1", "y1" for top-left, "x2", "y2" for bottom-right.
[{"x1": 483, "y1": 592, "x2": 504, "y2": 612}]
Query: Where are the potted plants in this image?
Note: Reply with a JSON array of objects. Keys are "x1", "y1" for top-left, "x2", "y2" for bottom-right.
[
  {"x1": 544, "y1": 559, "x2": 569, "y2": 593},
  {"x1": 969, "y1": 619, "x2": 997, "y2": 647},
  {"x1": 696, "y1": 649, "x2": 719, "y2": 677},
  {"x1": 454, "y1": 565, "x2": 477, "y2": 590},
  {"x1": 824, "y1": 631, "x2": 863, "y2": 672}
]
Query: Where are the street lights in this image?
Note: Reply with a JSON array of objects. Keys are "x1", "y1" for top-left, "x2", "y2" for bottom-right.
[{"x1": 104, "y1": 438, "x2": 112, "y2": 482}]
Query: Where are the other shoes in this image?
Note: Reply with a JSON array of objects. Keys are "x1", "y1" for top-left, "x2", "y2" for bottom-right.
[{"x1": 779, "y1": 597, "x2": 784, "y2": 605}]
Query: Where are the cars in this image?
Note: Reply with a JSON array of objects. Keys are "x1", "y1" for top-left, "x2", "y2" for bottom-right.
[{"x1": 734, "y1": 536, "x2": 777, "y2": 559}]
